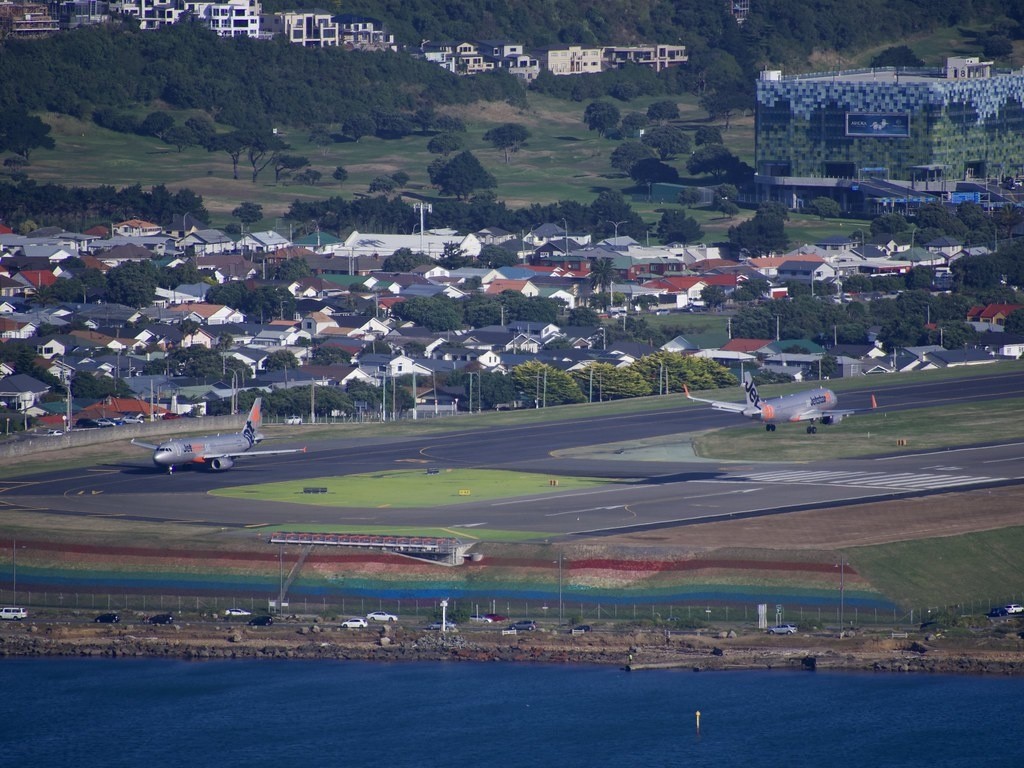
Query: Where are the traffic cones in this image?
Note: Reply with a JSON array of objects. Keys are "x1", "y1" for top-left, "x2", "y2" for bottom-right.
[{"x1": 871, "y1": 394, "x2": 877, "y2": 408}]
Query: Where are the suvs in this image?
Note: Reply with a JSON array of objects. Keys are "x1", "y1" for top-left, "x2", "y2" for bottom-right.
[
  {"x1": 509, "y1": 619, "x2": 536, "y2": 631},
  {"x1": 1004, "y1": 603, "x2": 1024, "y2": 614}
]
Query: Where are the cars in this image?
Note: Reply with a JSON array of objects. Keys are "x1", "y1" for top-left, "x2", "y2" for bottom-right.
[
  {"x1": 149, "y1": 614, "x2": 173, "y2": 624},
  {"x1": 990, "y1": 607, "x2": 1009, "y2": 617},
  {"x1": 486, "y1": 614, "x2": 510, "y2": 622},
  {"x1": 592, "y1": 286, "x2": 910, "y2": 320},
  {"x1": 427, "y1": 620, "x2": 457, "y2": 631},
  {"x1": 366, "y1": 612, "x2": 398, "y2": 623},
  {"x1": 284, "y1": 416, "x2": 303, "y2": 425},
  {"x1": 249, "y1": 615, "x2": 273, "y2": 626},
  {"x1": 767, "y1": 624, "x2": 798, "y2": 636},
  {"x1": 470, "y1": 614, "x2": 492, "y2": 624},
  {"x1": 341, "y1": 618, "x2": 368, "y2": 628},
  {"x1": 46, "y1": 410, "x2": 183, "y2": 438},
  {"x1": 570, "y1": 625, "x2": 592, "y2": 634},
  {"x1": 224, "y1": 609, "x2": 252, "y2": 616},
  {"x1": 95, "y1": 614, "x2": 120, "y2": 623}
]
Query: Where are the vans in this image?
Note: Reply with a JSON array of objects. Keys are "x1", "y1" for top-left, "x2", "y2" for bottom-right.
[{"x1": 0, "y1": 607, "x2": 27, "y2": 621}]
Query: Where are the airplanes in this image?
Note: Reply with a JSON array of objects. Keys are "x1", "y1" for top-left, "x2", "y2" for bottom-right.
[
  {"x1": 129, "y1": 396, "x2": 309, "y2": 478},
  {"x1": 683, "y1": 370, "x2": 855, "y2": 436}
]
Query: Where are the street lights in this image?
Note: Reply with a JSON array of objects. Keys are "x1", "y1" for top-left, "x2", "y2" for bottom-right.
[
  {"x1": 273, "y1": 543, "x2": 288, "y2": 622},
  {"x1": 552, "y1": 551, "x2": 567, "y2": 628},
  {"x1": 833, "y1": 552, "x2": 850, "y2": 633},
  {"x1": 3, "y1": 538, "x2": 27, "y2": 608}
]
